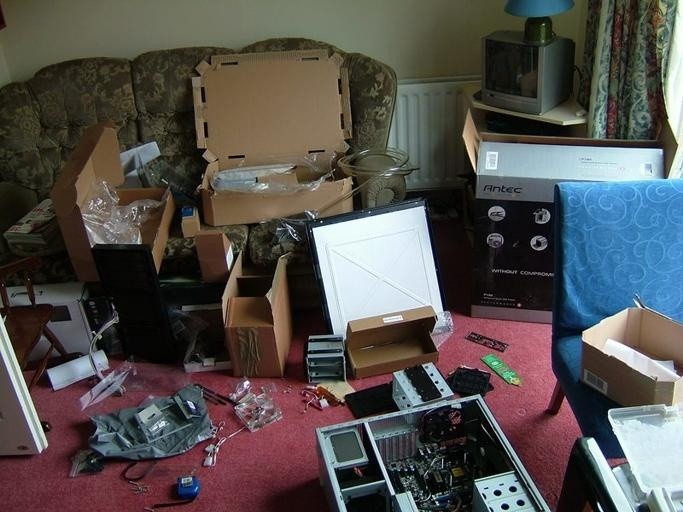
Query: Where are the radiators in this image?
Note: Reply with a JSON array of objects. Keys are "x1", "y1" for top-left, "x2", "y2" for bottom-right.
[{"x1": 387, "y1": 74, "x2": 482, "y2": 192}]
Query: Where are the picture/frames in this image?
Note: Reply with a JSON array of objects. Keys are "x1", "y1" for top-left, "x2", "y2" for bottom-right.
[{"x1": 304, "y1": 196, "x2": 450, "y2": 336}]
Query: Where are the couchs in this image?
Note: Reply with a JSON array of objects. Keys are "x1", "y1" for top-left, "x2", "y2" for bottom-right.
[{"x1": 0, "y1": 39, "x2": 406, "y2": 312}]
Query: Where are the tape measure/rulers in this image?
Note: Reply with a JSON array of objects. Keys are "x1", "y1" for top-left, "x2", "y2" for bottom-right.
[{"x1": 173, "y1": 475, "x2": 200, "y2": 497}]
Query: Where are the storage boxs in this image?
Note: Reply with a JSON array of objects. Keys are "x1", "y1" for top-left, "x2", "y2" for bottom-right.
[
  {"x1": 580, "y1": 294, "x2": 683, "y2": 408},
  {"x1": 191, "y1": 49, "x2": 356, "y2": 227},
  {"x1": 220, "y1": 251, "x2": 293, "y2": 378},
  {"x1": 51, "y1": 120, "x2": 177, "y2": 283},
  {"x1": 460, "y1": 110, "x2": 679, "y2": 325},
  {"x1": 347, "y1": 304, "x2": 439, "y2": 381}
]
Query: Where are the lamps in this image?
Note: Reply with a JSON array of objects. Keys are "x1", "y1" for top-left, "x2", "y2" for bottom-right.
[{"x1": 504, "y1": 0, "x2": 576, "y2": 47}]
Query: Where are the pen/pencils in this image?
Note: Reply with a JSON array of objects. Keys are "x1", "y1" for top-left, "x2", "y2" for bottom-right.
[{"x1": 193, "y1": 383, "x2": 238, "y2": 411}]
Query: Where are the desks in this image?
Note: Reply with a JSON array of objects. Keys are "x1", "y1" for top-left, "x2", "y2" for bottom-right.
[{"x1": 472, "y1": 88, "x2": 589, "y2": 139}]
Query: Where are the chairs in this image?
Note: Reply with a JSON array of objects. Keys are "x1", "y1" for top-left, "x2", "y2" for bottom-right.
[
  {"x1": 547, "y1": 179, "x2": 683, "y2": 465},
  {"x1": 0, "y1": 257, "x2": 85, "y2": 432}
]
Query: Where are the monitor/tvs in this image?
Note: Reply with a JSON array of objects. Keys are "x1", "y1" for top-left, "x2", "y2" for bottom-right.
[{"x1": 480, "y1": 30, "x2": 576, "y2": 116}]
